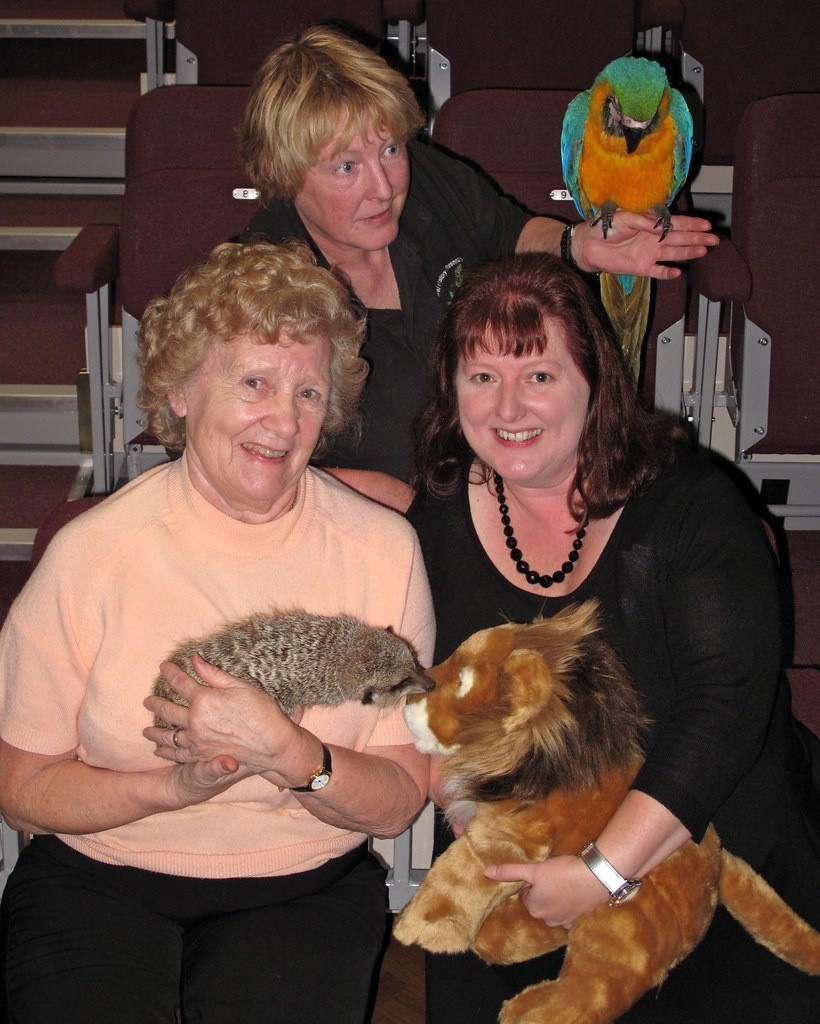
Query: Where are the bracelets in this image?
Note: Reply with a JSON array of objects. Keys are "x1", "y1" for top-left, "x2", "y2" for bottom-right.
[{"x1": 553, "y1": 223, "x2": 600, "y2": 280}]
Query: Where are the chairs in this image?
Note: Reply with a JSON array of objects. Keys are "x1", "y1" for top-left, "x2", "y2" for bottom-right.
[{"x1": 0, "y1": 0, "x2": 820, "y2": 1024}]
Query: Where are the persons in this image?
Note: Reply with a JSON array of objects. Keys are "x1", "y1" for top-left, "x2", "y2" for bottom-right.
[
  {"x1": 401, "y1": 251, "x2": 820, "y2": 1024},
  {"x1": 235, "y1": 23, "x2": 720, "y2": 520},
  {"x1": 0, "y1": 239, "x2": 437, "y2": 1023}
]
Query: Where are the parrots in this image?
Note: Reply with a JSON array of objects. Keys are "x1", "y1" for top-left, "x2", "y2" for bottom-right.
[{"x1": 561, "y1": 57, "x2": 694, "y2": 366}]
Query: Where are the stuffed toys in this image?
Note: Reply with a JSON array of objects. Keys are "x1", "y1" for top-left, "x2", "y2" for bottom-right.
[{"x1": 392, "y1": 597, "x2": 820, "y2": 1024}]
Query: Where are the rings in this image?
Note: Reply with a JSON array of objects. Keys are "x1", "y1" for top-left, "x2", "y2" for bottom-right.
[{"x1": 172, "y1": 728, "x2": 183, "y2": 747}]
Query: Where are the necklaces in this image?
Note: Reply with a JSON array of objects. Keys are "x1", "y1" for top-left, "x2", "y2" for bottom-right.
[{"x1": 490, "y1": 468, "x2": 590, "y2": 588}]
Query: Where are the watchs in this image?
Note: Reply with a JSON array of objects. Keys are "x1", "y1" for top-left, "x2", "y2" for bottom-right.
[
  {"x1": 287, "y1": 744, "x2": 332, "y2": 794},
  {"x1": 578, "y1": 839, "x2": 644, "y2": 908}
]
